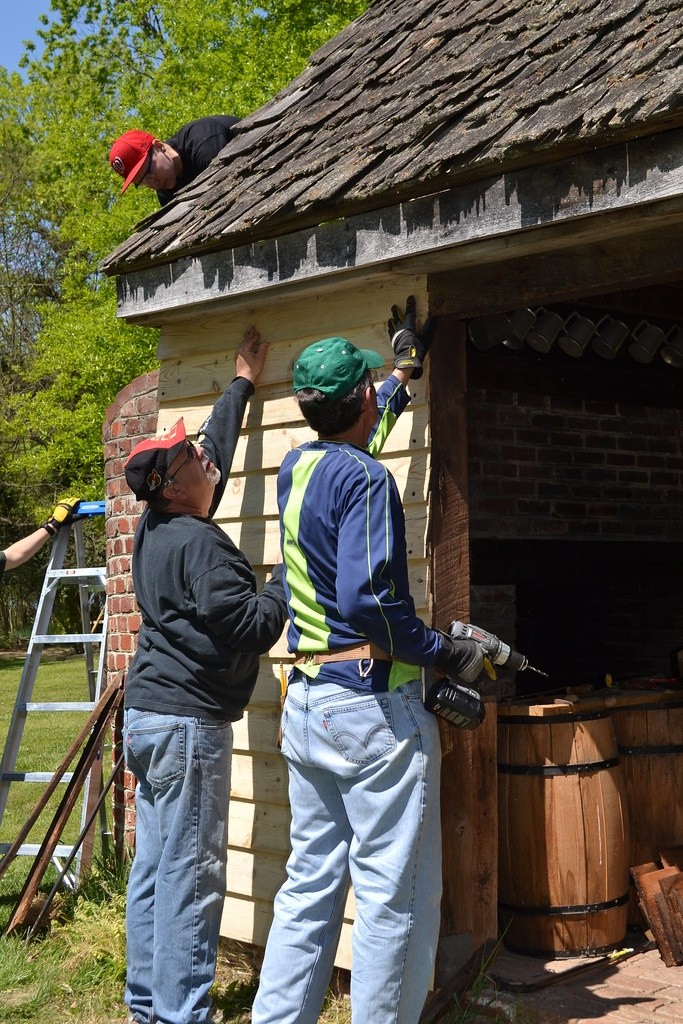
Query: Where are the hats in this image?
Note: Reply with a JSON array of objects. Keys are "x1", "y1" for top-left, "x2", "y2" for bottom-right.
[
  {"x1": 109, "y1": 131, "x2": 158, "y2": 197},
  {"x1": 124, "y1": 417, "x2": 186, "y2": 502},
  {"x1": 291, "y1": 337, "x2": 383, "y2": 410}
]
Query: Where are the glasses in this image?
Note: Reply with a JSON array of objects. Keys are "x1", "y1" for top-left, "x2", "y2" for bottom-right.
[
  {"x1": 161, "y1": 440, "x2": 197, "y2": 491},
  {"x1": 135, "y1": 148, "x2": 156, "y2": 190}
]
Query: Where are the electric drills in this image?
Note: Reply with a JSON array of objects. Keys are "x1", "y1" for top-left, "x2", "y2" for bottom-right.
[{"x1": 423, "y1": 617, "x2": 552, "y2": 730}]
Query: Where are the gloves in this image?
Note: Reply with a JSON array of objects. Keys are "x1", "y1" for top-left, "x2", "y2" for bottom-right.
[
  {"x1": 439, "y1": 629, "x2": 499, "y2": 684},
  {"x1": 47, "y1": 499, "x2": 90, "y2": 530},
  {"x1": 386, "y1": 295, "x2": 436, "y2": 379}
]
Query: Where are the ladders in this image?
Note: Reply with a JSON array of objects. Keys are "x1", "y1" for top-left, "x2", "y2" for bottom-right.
[{"x1": 0, "y1": 500, "x2": 107, "y2": 898}]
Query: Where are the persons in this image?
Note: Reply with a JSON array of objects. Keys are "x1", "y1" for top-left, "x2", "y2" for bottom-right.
[
  {"x1": 109, "y1": 115, "x2": 245, "y2": 208},
  {"x1": 0, "y1": 497, "x2": 87, "y2": 574},
  {"x1": 123, "y1": 324, "x2": 289, "y2": 1024},
  {"x1": 251, "y1": 293, "x2": 503, "y2": 1024}
]
